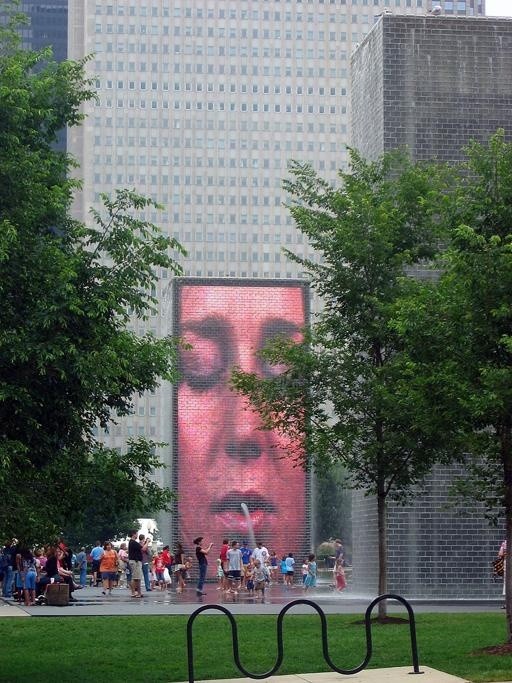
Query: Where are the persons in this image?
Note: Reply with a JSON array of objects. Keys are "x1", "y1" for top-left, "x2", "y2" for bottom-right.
[
  {"x1": 175, "y1": 283, "x2": 311, "y2": 580},
  {"x1": 498, "y1": 538, "x2": 507, "y2": 609}
]
